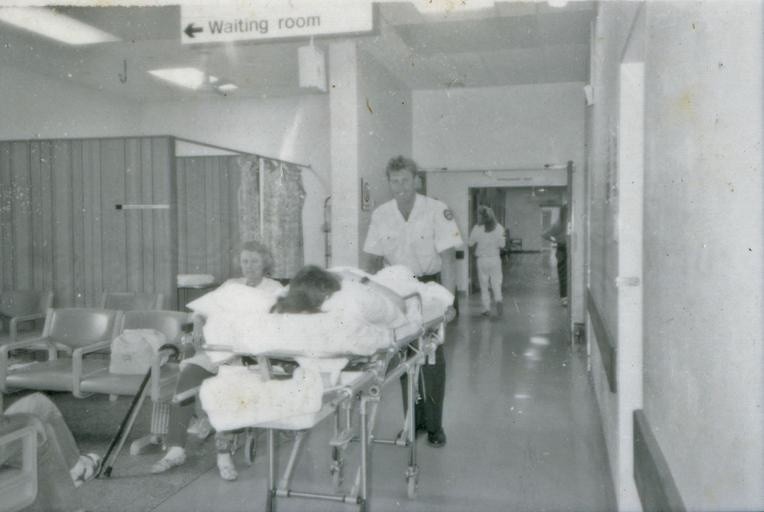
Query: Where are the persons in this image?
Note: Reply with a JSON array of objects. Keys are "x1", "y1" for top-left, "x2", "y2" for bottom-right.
[
  {"x1": 0, "y1": 387, "x2": 104, "y2": 488},
  {"x1": 269, "y1": 262, "x2": 408, "y2": 325},
  {"x1": 540, "y1": 203, "x2": 567, "y2": 306},
  {"x1": 467, "y1": 203, "x2": 507, "y2": 317},
  {"x1": 360, "y1": 156, "x2": 463, "y2": 449},
  {"x1": 149, "y1": 238, "x2": 281, "y2": 480}
]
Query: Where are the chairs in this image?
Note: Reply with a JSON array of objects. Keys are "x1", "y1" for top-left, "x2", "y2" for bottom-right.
[
  {"x1": 2, "y1": 307, "x2": 119, "y2": 393},
  {"x1": 2, "y1": 427, "x2": 37, "y2": 511},
  {"x1": 74, "y1": 311, "x2": 203, "y2": 400}
]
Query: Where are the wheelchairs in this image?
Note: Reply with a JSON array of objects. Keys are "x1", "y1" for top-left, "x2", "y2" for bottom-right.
[{"x1": 147, "y1": 308, "x2": 301, "y2": 471}]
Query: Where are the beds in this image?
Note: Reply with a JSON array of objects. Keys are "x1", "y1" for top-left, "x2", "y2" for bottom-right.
[{"x1": 197, "y1": 280, "x2": 459, "y2": 510}]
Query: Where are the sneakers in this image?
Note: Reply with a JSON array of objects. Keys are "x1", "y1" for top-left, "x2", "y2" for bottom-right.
[
  {"x1": 69, "y1": 452, "x2": 103, "y2": 488},
  {"x1": 428, "y1": 427, "x2": 446, "y2": 447},
  {"x1": 152, "y1": 446, "x2": 187, "y2": 473},
  {"x1": 216, "y1": 452, "x2": 238, "y2": 480}
]
